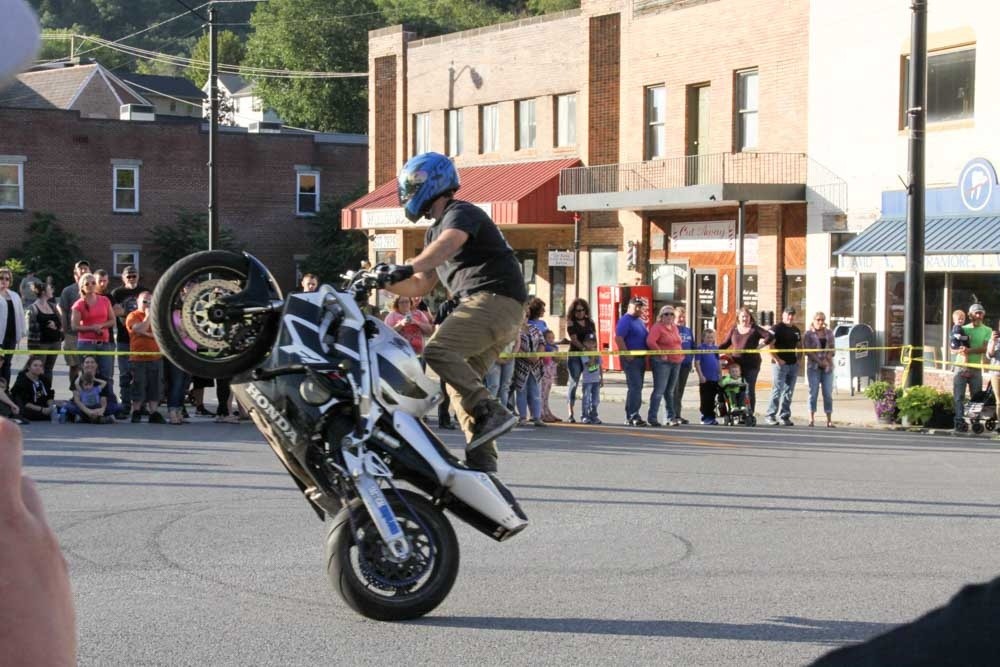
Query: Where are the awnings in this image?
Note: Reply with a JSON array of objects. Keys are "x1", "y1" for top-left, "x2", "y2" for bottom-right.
[{"x1": 341, "y1": 157, "x2": 581, "y2": 238}]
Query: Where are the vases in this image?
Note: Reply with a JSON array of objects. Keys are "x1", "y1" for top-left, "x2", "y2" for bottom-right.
[{"x1": 878, "y1": 416, "x2": 892, "y2": 425}]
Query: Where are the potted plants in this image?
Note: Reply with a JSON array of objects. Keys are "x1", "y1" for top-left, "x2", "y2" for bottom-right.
[{"x1": 895, "y1": 381, "x2": 969, "y2": 428}]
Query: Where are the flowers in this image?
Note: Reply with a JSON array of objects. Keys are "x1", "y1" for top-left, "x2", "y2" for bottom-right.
[{"x1": 863, "y1": 380, "x2": 895, "y2": 416}]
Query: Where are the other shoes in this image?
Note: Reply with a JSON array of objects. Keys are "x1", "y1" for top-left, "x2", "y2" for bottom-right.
[
  {"x1": 701, "y1": 417, "x2": 718, "y2": 425},
  {"x1": 666, "y1": 420, "x2": 679, "y2": 426},
  {"x1": 765, "y1": 415, "x2": 779, "y2": 425},
  {"x1": 74, "y1": 410, "x2": 242, "y2": 424},
  {"x1": 808, "y1": 420, "x2": 814, "y2": 426},
  {"x1": 624, "y1": 419, "x2": 647, "y2": 427},
  {"x1": 675, "y1": 416, "x2": 687, "y2": 424},
  {"x1": 519, "y1": 413, "x2": 601, "y2": 425},
  {"x1": 778, "y1": 417, "x2": 793, "y2": 426},
  {"x1": 827, "y1": 421, "x2": 836, "y2": 428},
  {"x1": 647, "y1": 421, "x2": 661, "y2": 427}
]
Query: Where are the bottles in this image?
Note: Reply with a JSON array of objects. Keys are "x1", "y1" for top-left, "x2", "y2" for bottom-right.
[
  {"x1": 50, "y1": 404, "x2": 58, "y2": 424},
  {"x1": 60, "y1": 402, "x2": 68, "y2": 423}
]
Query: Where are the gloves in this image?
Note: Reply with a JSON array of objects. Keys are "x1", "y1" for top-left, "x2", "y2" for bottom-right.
[{"x1": 376, "y1": 264, "x2": 414, "y2": 289}]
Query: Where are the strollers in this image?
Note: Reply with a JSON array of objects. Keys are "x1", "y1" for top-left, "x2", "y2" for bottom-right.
[{"x1": 713, "y1": 354, "x2": 757, "y2": 427}]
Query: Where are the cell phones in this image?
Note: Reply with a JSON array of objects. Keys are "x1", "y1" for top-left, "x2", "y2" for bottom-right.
[
  {"x1": 992, "y1": 327, "x2": 1000, "y2": 338},
  {"x1": 405, "y1": 314, "x2": 412, "y2": 321}
]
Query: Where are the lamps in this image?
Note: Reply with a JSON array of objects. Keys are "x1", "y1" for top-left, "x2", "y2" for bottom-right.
[{"x1": 626, "y1": 241, "x2": 639, "y2": 273}]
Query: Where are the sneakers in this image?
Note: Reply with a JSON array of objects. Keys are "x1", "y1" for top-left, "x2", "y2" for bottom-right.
[
  {"x1": 467, "y1": 399, "x2": 517, "y2": 452},
  {"x1": 458, "y1": 460, "x2": 497, "y2": 475}
]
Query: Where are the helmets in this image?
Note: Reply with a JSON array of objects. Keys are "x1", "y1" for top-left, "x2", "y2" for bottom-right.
[{"x1": 397, "y1": 151, "x2": 460, "y2": 223}]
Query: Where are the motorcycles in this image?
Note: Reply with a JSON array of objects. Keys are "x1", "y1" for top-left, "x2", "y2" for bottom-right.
[{"x1": 150, "y1": 248, "x2": 529, "y2": 622}]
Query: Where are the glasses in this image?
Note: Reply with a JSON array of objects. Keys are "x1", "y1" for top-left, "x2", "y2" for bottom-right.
[
  {"x1": 138, "y1": 299, "x2": 150, "y2": 305},
  {"x1": 84, "y1": 281, "x2": 96, "y2": 286},
  {"x1": 0, "y1": 276, "x2": 10, "y2": 282},
  {"x1": 664, "y1": 313, "x2": 674, "y2": 316},
  {"x1": 126, "y1": 274, "x2": 137, "y2": 279},
  {"x1": 576, "y1": 308, "x2": 585, "y2": 312}
]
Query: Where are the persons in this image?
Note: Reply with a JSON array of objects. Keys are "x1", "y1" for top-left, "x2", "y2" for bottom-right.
[
  {"x1": 950, "y1": 303, "x2": 1000, "y2": 431},
  {"x1": 0, "y1": 417, "x2": 76, "y2": 667},
  {"x1": 377, "y1": 153, "x2": 526, "y2": 475},
  {"x1": 0, "y1": 262, "x2": 246, "y2": 426},
  {"x1": 615, "y1": 296, "x2": 835, "y2": 427},
  {"x1": 300, "y1": 271, "x2": 604, "y2": 428}
]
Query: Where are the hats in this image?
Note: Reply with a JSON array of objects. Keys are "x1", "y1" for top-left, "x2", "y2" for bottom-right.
[
  {"x1": 969, "y1": 304, "x2": 985, "y2": 314},
  {"x1": 784, "y1": 306, "x2": 795, "y2": 313},
  {"x1": 630, "y1": 297, "x2": 642, "y2": 304},
  {"x1": 75, "y1": 260, "x2": 90, "y2": 268}
]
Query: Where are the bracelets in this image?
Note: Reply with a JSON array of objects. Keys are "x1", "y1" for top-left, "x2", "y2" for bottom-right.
[{"x1": 56, "y1": 327, "x2": 60, "y2": 331}]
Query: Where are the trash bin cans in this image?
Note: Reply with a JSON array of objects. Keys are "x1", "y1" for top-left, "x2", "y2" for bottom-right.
[{"x1": 832, "y1": 322, "x2": 876, "y2": 396}]
